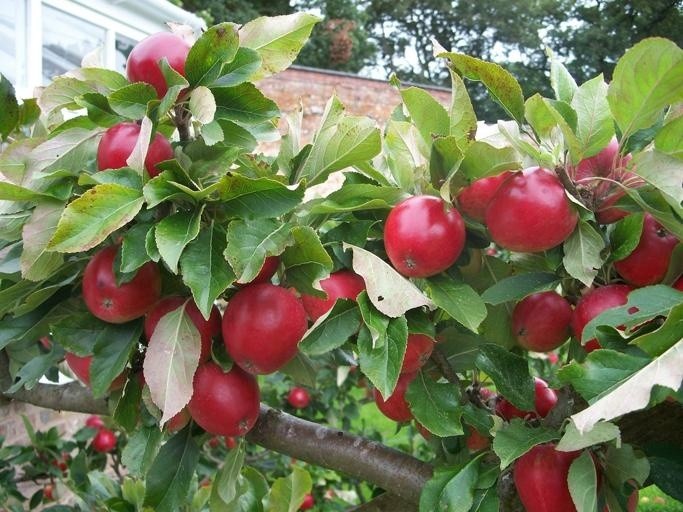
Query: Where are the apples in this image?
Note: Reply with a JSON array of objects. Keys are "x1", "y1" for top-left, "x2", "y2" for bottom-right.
[{"x1": 42, "y1": 32, "x2": 683, "y2": 512}]
